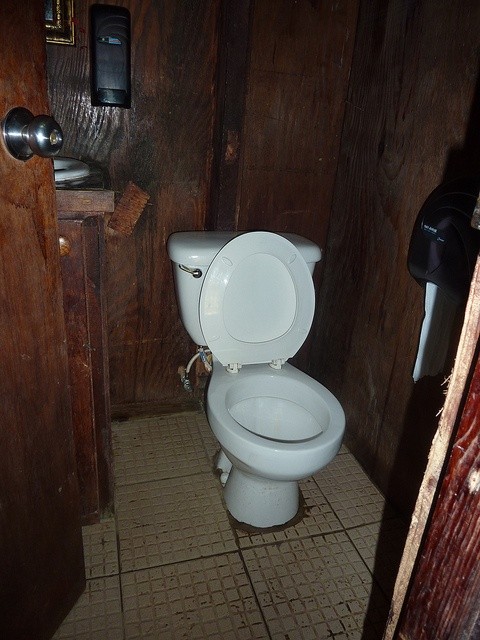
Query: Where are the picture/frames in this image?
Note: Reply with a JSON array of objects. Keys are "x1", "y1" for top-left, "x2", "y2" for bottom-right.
[{"x1": 46, "y1": 1, "x2": 75, "y2": 46}]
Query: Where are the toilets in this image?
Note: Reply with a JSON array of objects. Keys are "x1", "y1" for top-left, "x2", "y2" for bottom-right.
[{"x1": 167, "y1": 230, "x2": 347, "y2": 528}]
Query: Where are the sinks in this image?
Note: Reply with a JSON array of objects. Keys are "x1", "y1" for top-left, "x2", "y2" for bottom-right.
[{"x1": 54, "y1": 157, "x2": 90, "y2": 182}]
